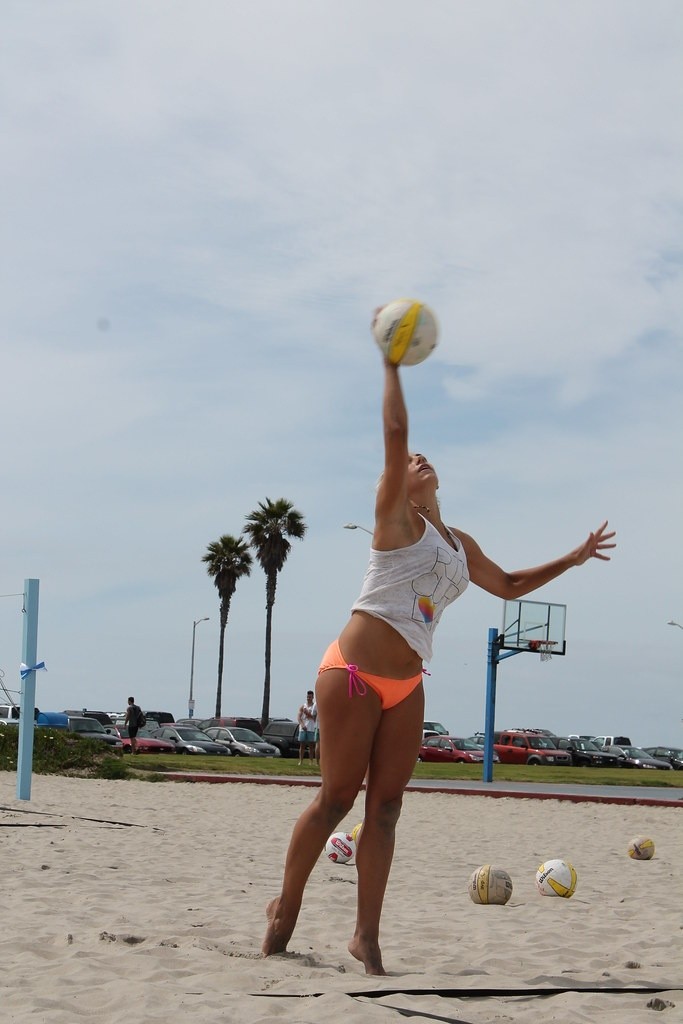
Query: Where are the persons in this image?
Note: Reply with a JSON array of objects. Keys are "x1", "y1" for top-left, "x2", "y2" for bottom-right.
[
  {"x1": 261, "y1": 302, "x2": 617, "y2": 975},
  {"x1": 123, "y1": 697, "x2": 140, "y2": 756},
  {"x1": 297, "y1": 691, "x2": 317, "y2": 765},
  {"x1": 311, "y1": 702, "x2": 320, "y2": 766}
]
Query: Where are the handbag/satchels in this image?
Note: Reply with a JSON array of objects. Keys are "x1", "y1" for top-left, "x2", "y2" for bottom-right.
[{"x1": 136, "y1": 712, "x2": 146, "y2": 729}]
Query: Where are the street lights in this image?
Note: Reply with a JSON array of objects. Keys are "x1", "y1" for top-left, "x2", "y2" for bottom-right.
[{"x1": 188, "y1": 617, "x2": 210, "y2": 718}]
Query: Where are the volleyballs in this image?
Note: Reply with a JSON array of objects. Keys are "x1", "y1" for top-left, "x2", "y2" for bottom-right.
[
  {"x1": 467, "y1": 863, "x2": 514, "y2": 906},
  {"x1": 351, "y1": 823, "x2": 364, "y2": 844},
  {"x1": 627, "y1": 835, "x2": 655, "y2": 861},
  {"x1": 534, "y1": 858, "x2": 579, "y2": 898},
  {"x1": 372, "y1": 297, "x2": 439, "y2": 367},
  {"x1": 324, "y1": 831, "x2": 356, "y2": 865}
]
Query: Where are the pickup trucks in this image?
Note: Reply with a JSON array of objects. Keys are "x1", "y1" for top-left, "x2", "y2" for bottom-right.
[{"x1": 492, "y1": 731, "x2": 572, "y2": 767}]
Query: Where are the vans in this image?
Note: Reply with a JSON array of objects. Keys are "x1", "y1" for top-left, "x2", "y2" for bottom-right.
[
  {"x1": 423, "y1": 721, "x2": 450, "y2": 736},
  {"x1": 423, "y1": 730, "x2": 439, "y2": 740},
  {"x1": 568, "y1": 733, "x2": 633, "y2": 750}
]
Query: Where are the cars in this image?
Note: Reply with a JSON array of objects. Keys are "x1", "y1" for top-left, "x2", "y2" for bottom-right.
[
  {"x1": 418, "y1": 736, "x2": 501, "y2": 765},
  {"x1": 149, "y1": 725, "x2": 231, "y2": 756},
  {"x1": 463, "y1": 736, "x2": 486, "y2": 748},
  {"x1": 549, "y1": 736, "x2": 619, "y2": 769},
  {"x1": 103, "y1": 724, "x2": 176, "y2": 754},
  {"x1": 601, "y1": 744, "x2": 675, "y2": 771},
  {"x1": 203, "y1": 726, "x2": 282, "y2": 758},
  {"x1": 639, "y1": 746, "x2": 683, "y2": 771},
  {"x1": 67, "y1": 715, "x2": 123, "y2": 756},
  {"x1": 62, "y1": 708, "x2": 204, "y2": 730}
]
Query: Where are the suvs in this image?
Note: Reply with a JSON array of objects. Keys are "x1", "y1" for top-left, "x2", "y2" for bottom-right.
[
  {"x1": 197, "y1": 716, "x2": 264, "y2": 736},
  {"x1": 0, "y1": 704, "x2": 41, "y2": 727},
  {"x1": 261, "y1": 721, "x2": 315, "y2": 758}
]
